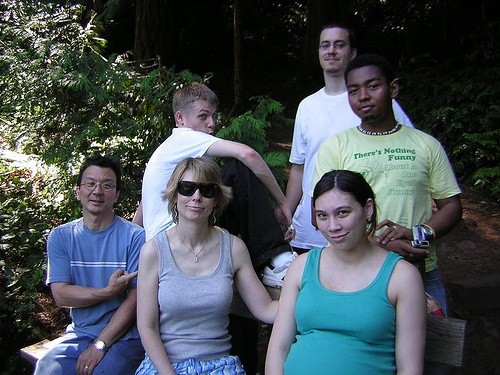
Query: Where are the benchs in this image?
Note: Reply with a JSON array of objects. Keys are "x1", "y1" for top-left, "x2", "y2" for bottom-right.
[{"x1": 20, "y1": 278, "x2": 468, "y2": 375}]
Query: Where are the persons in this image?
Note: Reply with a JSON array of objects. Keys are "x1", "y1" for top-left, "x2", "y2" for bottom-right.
[
  {"x1": 135, "y1": 157, "x2": 279, "y2": 375},
  {"x1": 309, "y1": 53, "x2": 464, "y2": 317},
  {"x1": 274, "y1": 23, "x2": 415, "y2": 254},
  {"x1": 264, "y1": 170, "x2": 427, "y2": 375},
  {"x1": 132, "y1": 83, "x2": 299, "y2": 288},
  {"x1": 34, "y1": 156, "x2": 146, "y2": 375}
]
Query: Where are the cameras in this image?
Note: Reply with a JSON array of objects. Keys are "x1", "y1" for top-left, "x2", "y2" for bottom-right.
[{"x1": 410, "y1": 241, "x2": 429, "y2": 256}]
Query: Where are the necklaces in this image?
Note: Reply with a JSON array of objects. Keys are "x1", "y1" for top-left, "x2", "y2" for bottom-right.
[{"x1": 182, "y1": 238, "x2": 208, "y2": 263}]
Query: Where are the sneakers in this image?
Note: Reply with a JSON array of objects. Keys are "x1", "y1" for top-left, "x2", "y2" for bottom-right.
[{"x1": 263, "y1": 251, "x2": 299, "y2": 288}]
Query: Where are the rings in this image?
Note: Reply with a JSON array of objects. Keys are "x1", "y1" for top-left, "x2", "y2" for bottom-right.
[
  {"x1": 393, "y1": 226, "x2": 396, "y2": 230},
  {"x1": 85, "y1": 365, "x2": 89, "y2": 368}
]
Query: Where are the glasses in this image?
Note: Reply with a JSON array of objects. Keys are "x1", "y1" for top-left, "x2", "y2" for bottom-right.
[
  {"x1": 178, "y1": 181, "x2": 222, "y2": 198},
  {"x1": 78, "y1": 180, "x2": 117, "y2": 191}
]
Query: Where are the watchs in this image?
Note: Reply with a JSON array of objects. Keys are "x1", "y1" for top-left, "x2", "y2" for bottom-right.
[{"x1": 93, "y1": 339, "x2": 109, "y2": 351}]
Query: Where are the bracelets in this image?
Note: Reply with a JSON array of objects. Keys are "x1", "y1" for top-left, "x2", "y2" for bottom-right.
[{"x1": 422, "y1": 224, "x2": 435, "y2": 240}]
